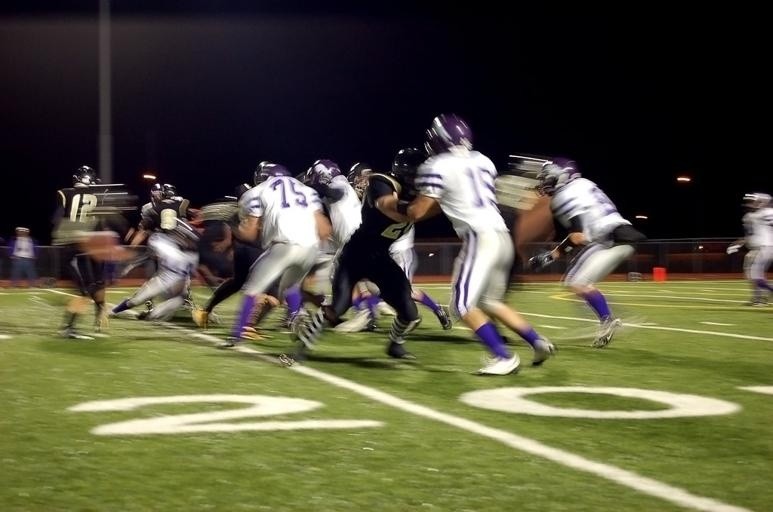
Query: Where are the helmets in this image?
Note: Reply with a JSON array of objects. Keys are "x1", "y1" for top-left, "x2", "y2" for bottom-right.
[
  {"x1": 393, "y1": 113, "x2": 472, "y2": 176},
  {"x1": 72, "y1": 166, "x2": 99, "y2": 186},
  {"x1": 536, "y1": 158, "x2": 581, "y2": 197},
  {"x1": 254, "y1": 159, "x2": 372, "y2": 198},
  {"x1": 149, "y1": 182, "x2": 183, "y2": 214}
]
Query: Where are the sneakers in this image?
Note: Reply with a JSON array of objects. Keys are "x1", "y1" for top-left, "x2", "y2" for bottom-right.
[
  {"x1": 478, "y1": 338, "x2": 553, "y2": 375},
  {"x1": 434, "y1": 307, "x2": 452, "y2": 330}
]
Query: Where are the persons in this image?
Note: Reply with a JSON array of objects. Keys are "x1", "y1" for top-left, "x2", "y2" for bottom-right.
[
  {"x1": 53, "y1": 115, "x2": 646, "y2": 375},
  {"x1": 728, "y1": 191, "x2": 772, "y2": 308},
  {"x1": 7, "y1": 226, "x2": 39, "y2": 288}
]
